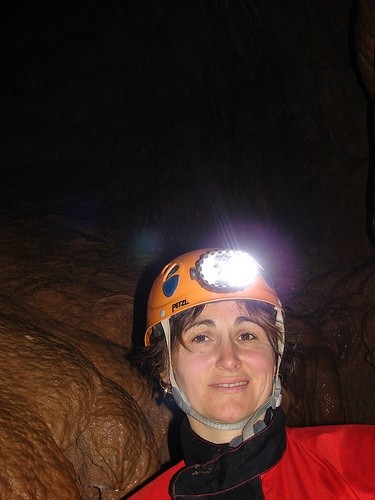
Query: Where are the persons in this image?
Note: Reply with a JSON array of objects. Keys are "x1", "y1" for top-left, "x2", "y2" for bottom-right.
[{"x1": 122, "y1": 247, "x2": 375, "y2": 500}]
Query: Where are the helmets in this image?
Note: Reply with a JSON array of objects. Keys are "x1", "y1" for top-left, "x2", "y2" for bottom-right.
[{"x1": 144, "y1": 248, "x2": 285, "y2": 348}]
